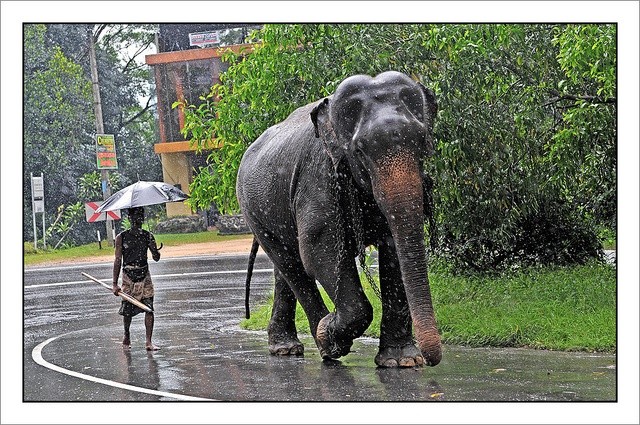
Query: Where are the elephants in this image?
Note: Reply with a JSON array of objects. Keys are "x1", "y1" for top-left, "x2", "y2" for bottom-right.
[{"x1": 234, "y1": 69, "x2": 442, "y2": 369}]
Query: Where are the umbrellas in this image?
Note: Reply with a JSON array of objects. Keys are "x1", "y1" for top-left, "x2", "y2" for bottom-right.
[{"x1": 94, "y1": 172, "x2": 191, "y2": 252}]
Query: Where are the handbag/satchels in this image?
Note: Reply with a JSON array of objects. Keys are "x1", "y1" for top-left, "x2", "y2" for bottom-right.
[{"x1": 128, "y1": 269, "x2": 146, "y2": 283}]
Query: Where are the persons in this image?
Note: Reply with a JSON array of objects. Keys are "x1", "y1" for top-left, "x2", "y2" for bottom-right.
[{"x1": 111, "y1": 206, "x2": 162, "y2": 352}]
